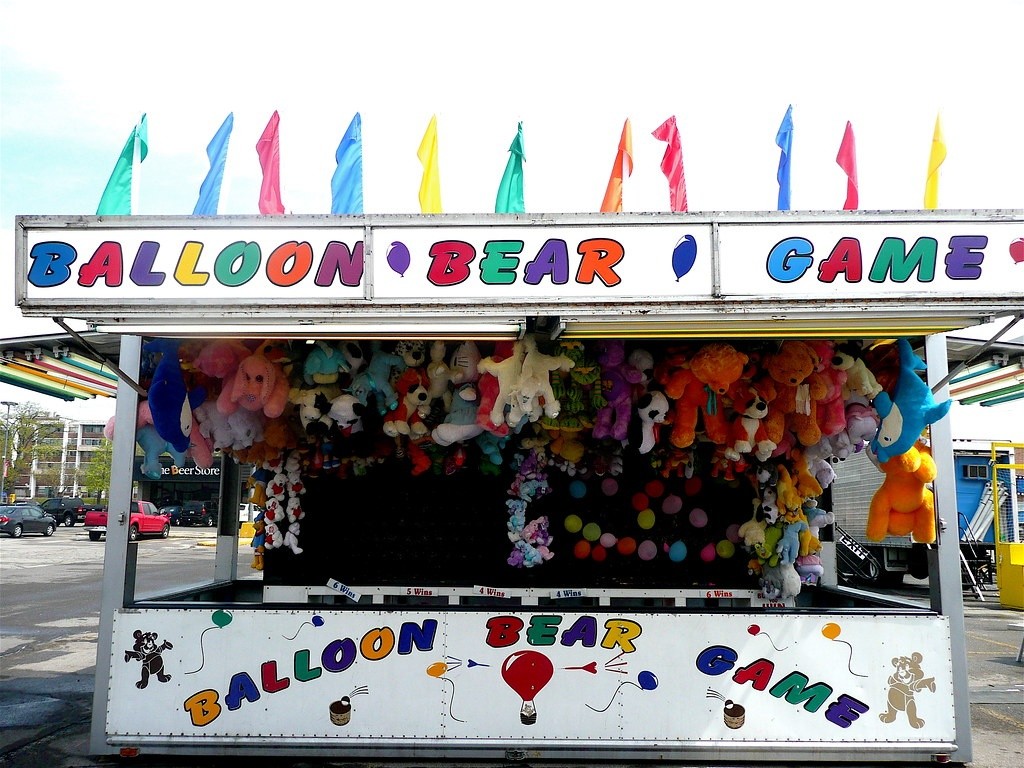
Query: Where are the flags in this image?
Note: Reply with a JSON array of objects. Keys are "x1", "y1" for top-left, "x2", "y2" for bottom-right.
[
  {"x1": 192, "y1": 112, "x2": 233, "y2": 217},
  {"x1": 495, "y1": 121, "x2": 527, "y2": 214},
  {"x1": 837, "y1": 121, "x2": 859, "y2": 210},
  {"x1": 94, "y1": 113, "x2": 149, "y2": 215},
  {"x1": 417, "y1": 115, "x2": 443, "y2": 215},
  {"x1": 255, "y1": 111, "x2": 287, "y2": 215},
  {"x1": 775, "y1": 105, "x2": 793, "y2": 210},
  {"x1": 652, "y1": 115, "x2": 689, "y2": 213},
  {"x1": 923, "y1": 113, "x2": 948, "y2": 211},
  {"x1": 330, "y1": 113, "x2": 365, "y2": 214},
  {"x1": 600, "y1": 119, "x2": 634, "y2": 212}
]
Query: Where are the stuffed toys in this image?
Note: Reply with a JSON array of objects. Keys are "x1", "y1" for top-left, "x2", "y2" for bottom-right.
[
  {"x1": 286, "y1": 471, "x2": 306, "y2": 497},
  {"x1": 263, "y1": 498, "x2": 285, "y2": 525},
  {"x1": 737, "y1": 479, "x2": 835, "y2": 599},
  {"x1": 286, "y1": 498, "x2": 305, "y2": 523},
  {"x1": 505, "y1": 499, "x2": 555, "y2": 569},
  {"x1": 535, "y1": 474, "x2": 552, "y2": 498},
  {"x1": 867, "y1": 427, "x2": 937, "y2": 544},
  {"x1": 266, "y1": 474, "x2": 288, "y2": 501},
  {"x1": 520, "y1": 480, "x2": 539, "y2": 502},
  {"x1": 508, "y1": 473, "x2": 526, "y2": 497},
  {"x1": 248, "y1": 467, "x2": 277, "y2": 507},
  {"x1": 250, "y1": 521, "x2": 302, "y2": 570},
  {"x1": 136, "y1": 340, "x2": 951, "y2": 479}
]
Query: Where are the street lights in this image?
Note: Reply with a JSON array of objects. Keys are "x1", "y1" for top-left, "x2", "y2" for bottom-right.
[{"x1": 0, "y1": 400, "x2": 18, "y2": 503}]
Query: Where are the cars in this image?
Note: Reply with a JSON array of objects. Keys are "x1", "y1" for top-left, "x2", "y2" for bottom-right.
[
  {"x1": 239, "y1": 504, "x2": 263, "y2": 528},
  {"x1": 156, "y1": 500, "x2": 185, "y2": 526},
  {"x1": 0, "y1": 505, "x2": 57, "y2": 537}
]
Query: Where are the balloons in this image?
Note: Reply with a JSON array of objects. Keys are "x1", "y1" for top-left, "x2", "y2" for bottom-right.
[
  {"x1": 563, "y1": 479, "x2": 734, "y2": 562},
  {"x1": 728, "y1": 524, "x2": 741, "y2": 544}
]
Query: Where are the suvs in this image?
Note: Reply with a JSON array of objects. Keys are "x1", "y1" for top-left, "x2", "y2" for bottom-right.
[{"x1": 181, "y1": 500, "x2": 219, "y2": 526}]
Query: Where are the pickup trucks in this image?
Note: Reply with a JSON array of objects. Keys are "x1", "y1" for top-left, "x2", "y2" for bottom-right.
[
  {"x1": 39, "y1": 497, "x2": 104, "y2": 527},
  {"x1": 83, "y1": 500, "x2": 173, "y2": 541}
]
